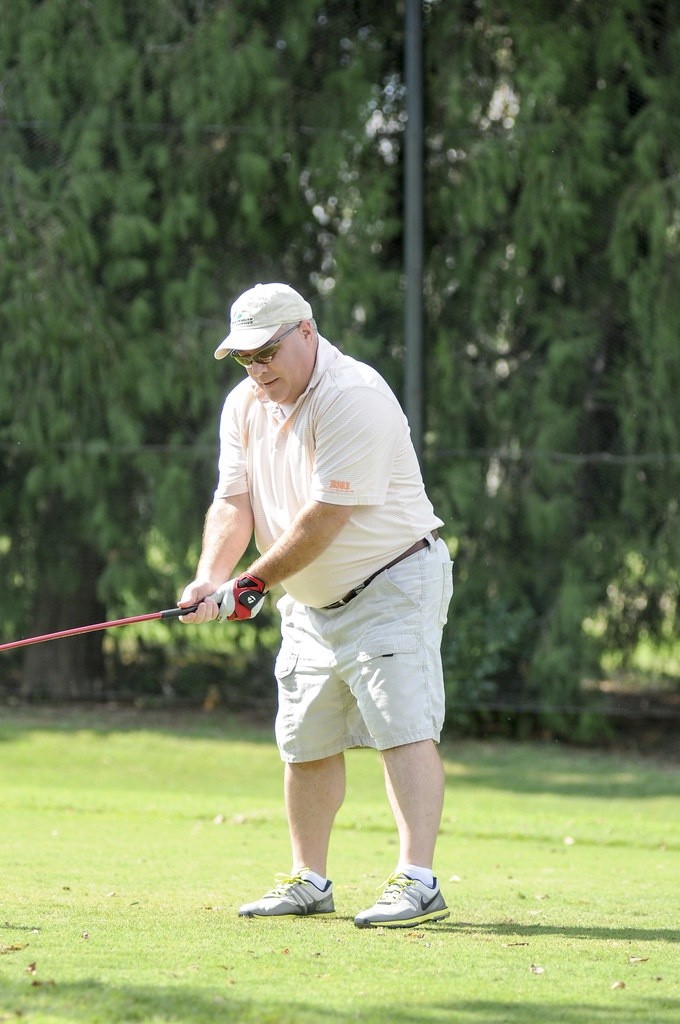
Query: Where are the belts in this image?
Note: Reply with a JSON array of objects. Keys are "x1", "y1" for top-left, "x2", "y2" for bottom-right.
[{"x1": 322, "y1": 528, "x2": 439, "y2": 609}]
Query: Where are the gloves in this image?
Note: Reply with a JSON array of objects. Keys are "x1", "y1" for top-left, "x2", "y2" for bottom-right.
[{"x1": 205, "y1": 571, "x2": 270, "y2": 624}]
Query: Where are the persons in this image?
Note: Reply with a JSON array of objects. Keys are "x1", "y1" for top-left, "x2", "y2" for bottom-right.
[{"x1": 178, "y1": 283, "x2": 453, "y2": 929}]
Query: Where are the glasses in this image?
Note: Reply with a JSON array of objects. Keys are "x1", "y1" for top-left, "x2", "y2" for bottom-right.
[{"x1": 230, "y1": 321, "x2": 311, "y2": 369}]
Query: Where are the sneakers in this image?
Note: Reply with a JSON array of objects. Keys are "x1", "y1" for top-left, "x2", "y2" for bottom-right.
[
  {"x1": 354, "y1": 872, "x2": 450, "y2": 929},
  {"x1": 237, "y1": 867, "x2": 335, "y2": 919}
]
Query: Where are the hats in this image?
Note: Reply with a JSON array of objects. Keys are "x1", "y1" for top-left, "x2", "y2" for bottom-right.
[{"x1": 213, "y1": 283, "x2": 313, "y2": 360}]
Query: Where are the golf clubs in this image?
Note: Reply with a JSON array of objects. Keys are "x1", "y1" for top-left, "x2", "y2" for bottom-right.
[{"x1": 0, "y1": 602, "x2": 222, "y2": 651}]
